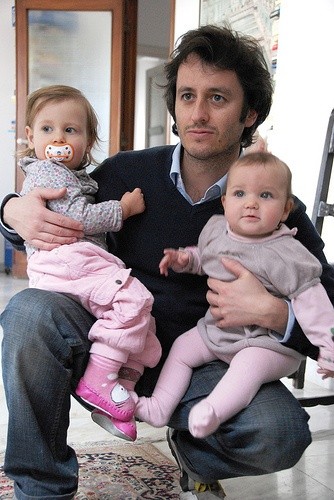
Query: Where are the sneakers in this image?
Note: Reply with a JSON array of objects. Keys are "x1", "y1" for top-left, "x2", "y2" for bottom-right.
[{"x1": 162, "y1": 429, "x2": 227, "y2": 499}]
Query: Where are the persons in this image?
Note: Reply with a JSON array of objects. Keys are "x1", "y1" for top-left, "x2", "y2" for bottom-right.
[
  {"x1": 0, "y1": 20, "x2": 334, "y2": 500},
  {"x1": 128, "y1": 153, "x2": 334, "y2": 438},
  {"x1": 24, "y1": 85, "x2": 162, "y2": 442}
]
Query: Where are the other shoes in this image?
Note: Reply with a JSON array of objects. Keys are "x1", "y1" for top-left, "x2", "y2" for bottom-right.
[{"x1": 75, "y1": 377, "x2": 138, "y2": 442}]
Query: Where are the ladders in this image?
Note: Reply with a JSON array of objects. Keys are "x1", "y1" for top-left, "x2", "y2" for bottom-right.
[{"x1": 292, "y1": 110, "x2": 334, "y2": 414}]
